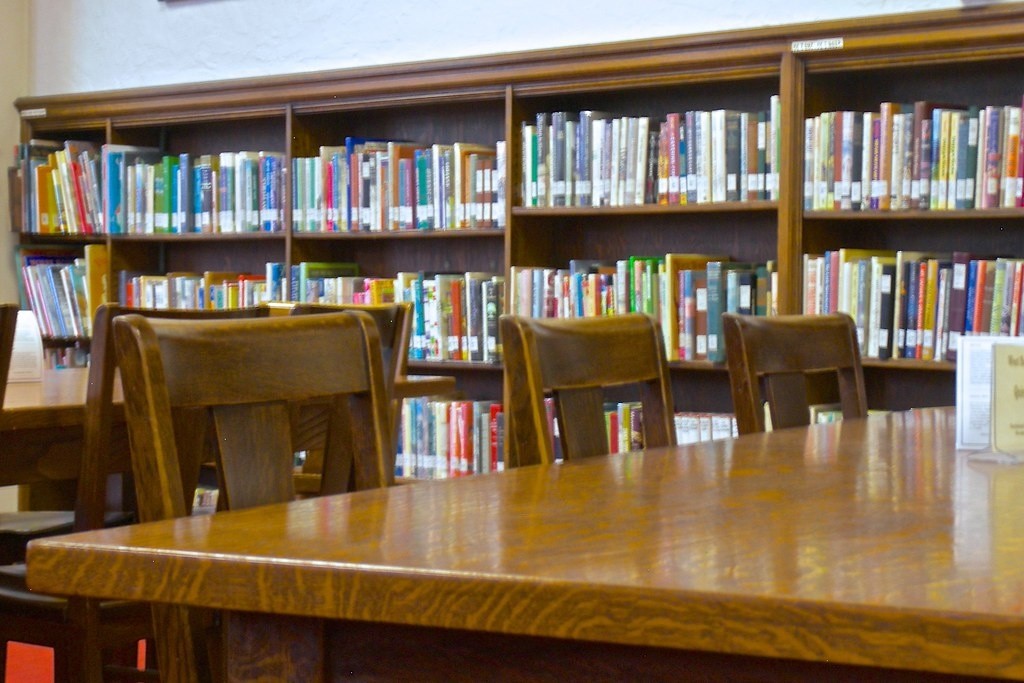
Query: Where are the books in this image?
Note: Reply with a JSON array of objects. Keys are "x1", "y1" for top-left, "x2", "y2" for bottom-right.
[{"x1": 8, "y1": 103, "x2": 1024, "y2": 510}]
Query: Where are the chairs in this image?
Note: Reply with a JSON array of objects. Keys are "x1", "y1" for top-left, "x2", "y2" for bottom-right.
[{"x1": 0, "y1": 290, "x2": 871, "y2": 683}]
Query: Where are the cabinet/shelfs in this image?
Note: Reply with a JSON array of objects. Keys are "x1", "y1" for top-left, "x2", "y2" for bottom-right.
[{"x1": 14, "y1": 0, "x2": 1024, "y2": 481}]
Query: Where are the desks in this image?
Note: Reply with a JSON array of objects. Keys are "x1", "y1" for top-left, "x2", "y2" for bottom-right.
[
  {"x1": 0, "y1": 369, "x2": 130, "y2": 488},
  {"x1": 26, "y1": 406, "x2": 1024, "y2": 682}
]
